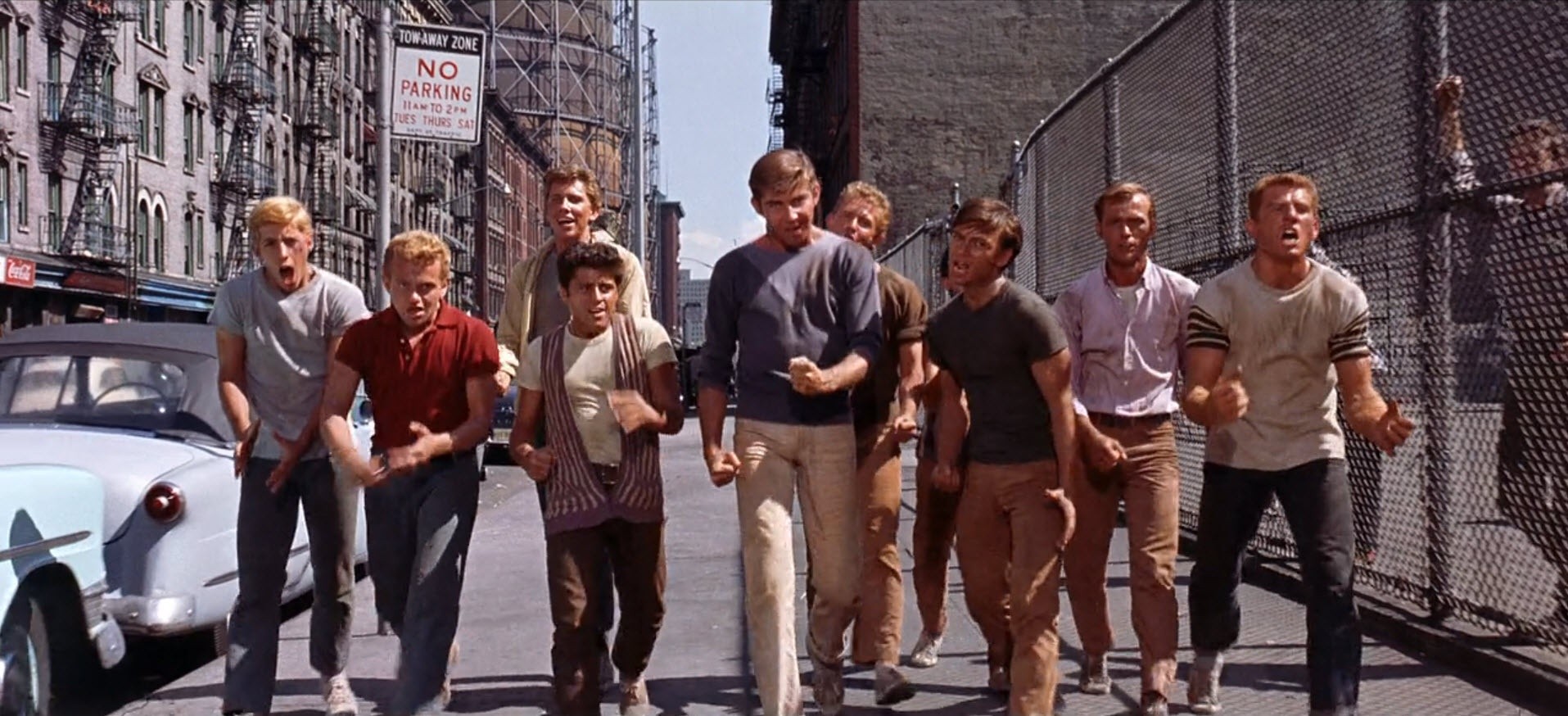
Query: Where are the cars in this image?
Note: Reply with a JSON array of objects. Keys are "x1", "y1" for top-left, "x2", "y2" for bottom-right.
[{"x1": 0, "y1": 314, "x2": 367, "y2": 716}]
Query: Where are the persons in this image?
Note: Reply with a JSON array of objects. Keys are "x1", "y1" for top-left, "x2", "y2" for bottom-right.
[
  {"x1": 698, "y1": 150, "x2": 881, "y2": 715},
  {"x1": 320, "y1": 231, "x2": 500, "y2": 716},
  {"x1": 826, "y1": 181, "x2": 929, "y2": 704},
  {"x1": 494, "y1": 165, "x2": 654, "y2": 681},
  {"x1": 1182, "y1": 172, "x2": 1412, "y2": 715},
  {"x1": 207, "y1": 197, "x2": 369, "y2": 716},
  {"x1": 510, "y1": 245, "x2": 684, "y2": 716},
  {"x1": 1049, "y1": 183, "x2": 1202, "y2": 716},
  {"x1": 926, "y1": 198, "x2": 1081, "y2": 715},
  {"x1": 908, "y1": 252, "x2": 1016, "y2": 666},
  {"x1": 1422, "y1": 75, "x2": 1568, "y2": 648}
]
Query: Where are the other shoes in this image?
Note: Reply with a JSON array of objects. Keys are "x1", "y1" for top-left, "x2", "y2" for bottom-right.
[
  {"x1": 989, "y1": 666, "x2": 1009, "y2": 689},
  {"x1": 1188, "y1": 663, "x2": 1223, "y2": 712},
  {"x1": 620, "y1": 675, "x2": 650, "y2": 716},
  {"x1": 599, "y1": 634, "x2": 615, "y2": 692},
  {"x1": 1080, "y1": 650, "x2": 1112, "y2": 695},
  {"x1": 1140, "y1": 694, "x2": 1169, "y2": 716}
]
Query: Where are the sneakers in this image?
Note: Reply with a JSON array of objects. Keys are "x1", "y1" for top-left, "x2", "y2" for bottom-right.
[
  {"x1": 321, "y1": 671, "x2": 357, "y2": 715},
  {"x1": 911, "y1": 632, "x2": 942, "y2": 667},
  {"x1": 876, "y1": 666, "x2": 915, "y2": 705},
  {"x1": 804, "y1": 637, "x2": 846, "y2": 715}
]
